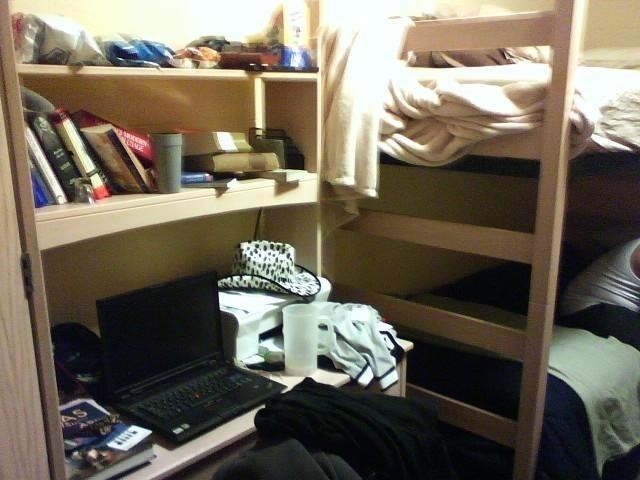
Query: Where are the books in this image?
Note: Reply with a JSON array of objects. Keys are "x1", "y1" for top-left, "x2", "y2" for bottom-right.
[
  {"x1": 179, "y1": 125, "x2": 297, "y2": 187},
  {"x1": 56, "y1": 398, "x2": 160, "y2": 479},
  {"x1": 20, "y1": 106, "x2": 154, "y2": 208}
]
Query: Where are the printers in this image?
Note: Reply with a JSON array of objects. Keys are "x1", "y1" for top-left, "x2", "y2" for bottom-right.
[{"x1": 218, "y1": 275, "x2": 333, "y2": 365}]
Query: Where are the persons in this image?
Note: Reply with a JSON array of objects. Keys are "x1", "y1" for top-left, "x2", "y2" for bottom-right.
[{"x1": 555, "y1": 234, "x2": 640, "y2": 356}]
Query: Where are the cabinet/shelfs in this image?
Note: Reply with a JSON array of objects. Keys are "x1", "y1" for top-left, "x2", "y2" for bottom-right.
[{"x1": 0, "y1": 0, "x2": 414, "y2": 480}]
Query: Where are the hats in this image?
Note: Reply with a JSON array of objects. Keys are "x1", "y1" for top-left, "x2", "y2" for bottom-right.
[{"x1": 217, "y1": 240, "x2": 321, "y2": 296}]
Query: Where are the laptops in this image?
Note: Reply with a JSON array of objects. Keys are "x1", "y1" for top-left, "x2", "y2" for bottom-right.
[{"x1": 96, "y1": 272, "x2": 288, "y2": 444}]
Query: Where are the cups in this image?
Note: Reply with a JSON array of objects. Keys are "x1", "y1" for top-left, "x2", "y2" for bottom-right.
[
  {"x1": 149, "y1": 131, "x2": 183, "y2": 194},
  {"x1": 74, "y1": 176, "x2": 97, "y2": 205},
  {"x1": 279, "y1": 305, "x2": 335, "y2": 377}
]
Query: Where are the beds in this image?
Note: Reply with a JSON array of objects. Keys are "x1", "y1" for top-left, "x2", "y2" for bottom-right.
[{"x1": 327, "y1": 0, "x2": 640, "y2": 480}]
